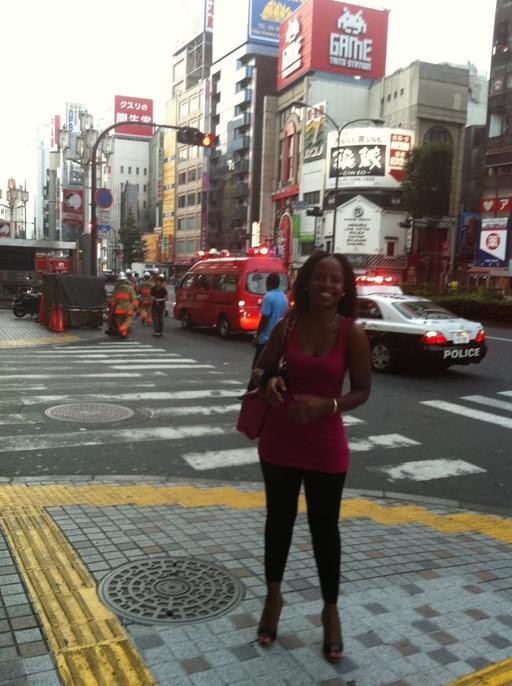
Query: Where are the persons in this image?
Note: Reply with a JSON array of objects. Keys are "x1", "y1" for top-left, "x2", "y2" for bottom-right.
[
  {"x1": 245, "y1": 252, "x2": 371, "y2": 663},
  {"x1": 104, "y1": 268, "x2": 169, "y2": 337},
  {"x1": 250, "y1": 271, "x2": 288, "y2": 372}
]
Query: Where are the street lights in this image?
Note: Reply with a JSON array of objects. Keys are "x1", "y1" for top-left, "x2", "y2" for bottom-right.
[
  {"x1": 0, "y1": 177, "x2": 31, "y2": 239},
  {"x1": 301, "y1": 203, "x2": 325, "y2": 250},
  {"x1": 55, "y1": 107, "x2": 112, "y2": 274},
  {"x1": 290, "y1": 100, "x2": 387, "y2": 254}
]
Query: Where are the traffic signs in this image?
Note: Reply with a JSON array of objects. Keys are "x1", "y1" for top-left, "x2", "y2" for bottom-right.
[{"x1": 34, "y1": 294, "x2": 67, "y2": 335}]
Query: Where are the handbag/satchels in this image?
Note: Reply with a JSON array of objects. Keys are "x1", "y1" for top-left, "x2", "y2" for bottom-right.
[{"x1": 236, "y1": 388, "x2": 280, "y2": 440}]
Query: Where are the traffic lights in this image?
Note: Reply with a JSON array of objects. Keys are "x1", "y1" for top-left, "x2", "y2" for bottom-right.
[{"x1": 175, "y1": 126, "x2": 221, "y2": 150}]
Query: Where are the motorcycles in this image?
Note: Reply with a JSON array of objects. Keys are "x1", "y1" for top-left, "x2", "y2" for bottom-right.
[{"x1": 12, "y1": 286, "x2": 43, "y2": 320}]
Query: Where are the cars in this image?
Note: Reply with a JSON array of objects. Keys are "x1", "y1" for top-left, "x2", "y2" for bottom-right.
[{"x1": 347, "y1": 274, "x2": 494, "y2": 376}]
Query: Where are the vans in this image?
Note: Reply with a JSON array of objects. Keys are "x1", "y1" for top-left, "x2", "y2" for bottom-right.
[{"x1": 168, "y1": 246, "x2": 299, "y2": 340}]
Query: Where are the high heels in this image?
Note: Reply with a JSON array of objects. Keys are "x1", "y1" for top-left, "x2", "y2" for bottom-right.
[
  {"x1": 322, "y1": 604, "x2": 343, "y2": 663},
  {"x1": 257, "y1": 594, "x2": 284, "y2": 646}
]
